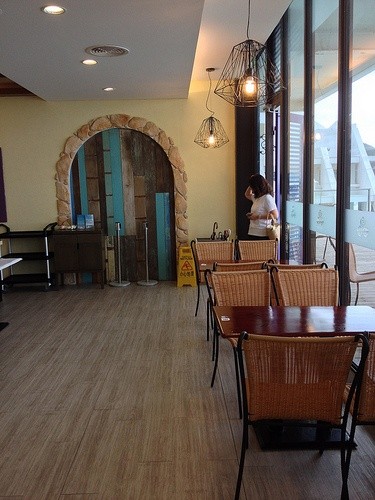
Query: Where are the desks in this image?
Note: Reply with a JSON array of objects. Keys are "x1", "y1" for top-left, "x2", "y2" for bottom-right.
[
  {"x1": 198, "y1": 260, "x2": 302, "y2": 273},
  {"x1": 0, "y1": 258, "x2": 23, "y2": 332},
  {"x1": 213, "y1": 305, "x2": 375, "y2": 452}
]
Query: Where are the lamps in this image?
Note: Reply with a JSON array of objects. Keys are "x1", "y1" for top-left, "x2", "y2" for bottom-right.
[
  {"x1": 214, "y1": 0, "x2": 287, "y2": 109},
  {"x1": 194, "y1": 68, "x2": 229, "y2": 149}
]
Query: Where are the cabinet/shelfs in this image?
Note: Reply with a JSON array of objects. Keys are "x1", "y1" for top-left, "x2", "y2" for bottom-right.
[
  {"x1": 52, "y1": 228, "x2": 107, "y2": 289},
  {"x1": 0, "y1": 222, "x2": 57, "y2": 291}
]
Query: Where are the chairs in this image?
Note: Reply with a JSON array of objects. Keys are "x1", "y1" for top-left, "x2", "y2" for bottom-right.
[{"x1": 191, "y1": 238, "x2": 375, "y2": 500}]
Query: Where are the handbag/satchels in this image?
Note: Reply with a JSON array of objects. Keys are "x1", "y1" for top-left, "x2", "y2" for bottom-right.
[{"x1": 266, "y1": 224, "x2": 280, "y2": 241}]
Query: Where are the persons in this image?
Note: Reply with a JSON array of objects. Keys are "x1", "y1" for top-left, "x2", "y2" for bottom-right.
[{"x1": 245, "y1": 174, "x2": 278, "y2": 240}]
